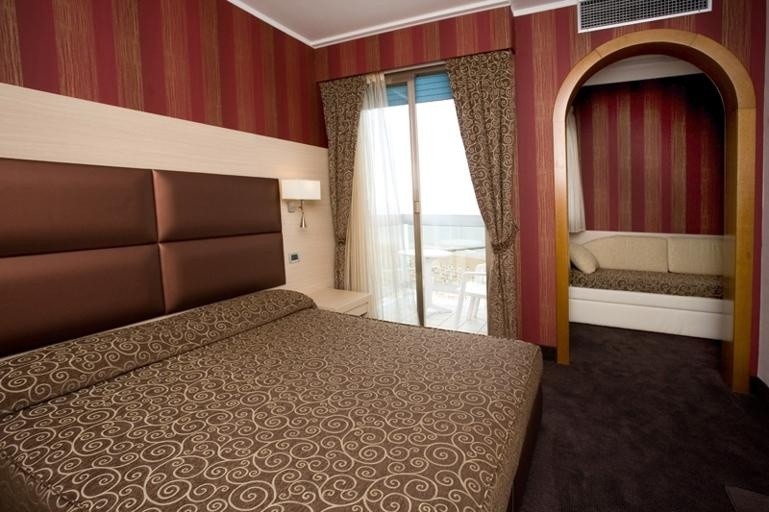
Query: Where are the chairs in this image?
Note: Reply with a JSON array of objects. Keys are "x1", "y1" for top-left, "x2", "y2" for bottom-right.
[{"x1": 454, "y1": 264, "x2": 489, "y2": 328}]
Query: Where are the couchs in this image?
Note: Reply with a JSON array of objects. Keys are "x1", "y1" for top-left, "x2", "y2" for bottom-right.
[{"x1": 569, "y1": 229, "x2": 724, "y2": 341}]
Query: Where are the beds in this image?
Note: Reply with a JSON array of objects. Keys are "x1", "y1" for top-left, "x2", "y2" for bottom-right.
[{"x1": 0, "y1": 155, "x2": 545, "y2": 511}]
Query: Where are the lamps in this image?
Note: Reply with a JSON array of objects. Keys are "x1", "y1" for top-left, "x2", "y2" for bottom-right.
[{"x1": 280, "y1": 179, "x2": 322, "y2": 229}]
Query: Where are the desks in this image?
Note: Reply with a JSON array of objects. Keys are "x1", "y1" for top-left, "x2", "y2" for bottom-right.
[{"x1": 398, "y1": 247, "x2": 453, "y2": 318}]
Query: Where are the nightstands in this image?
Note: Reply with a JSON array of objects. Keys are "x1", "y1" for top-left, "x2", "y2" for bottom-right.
[{"x1": 306, "y1": 287, "x2": 373, "y2": 318}]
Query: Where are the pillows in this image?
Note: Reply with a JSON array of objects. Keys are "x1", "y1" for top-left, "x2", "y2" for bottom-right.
[{"x1": 568, "y1": 242, "x2": 599, "y2": 275}]
